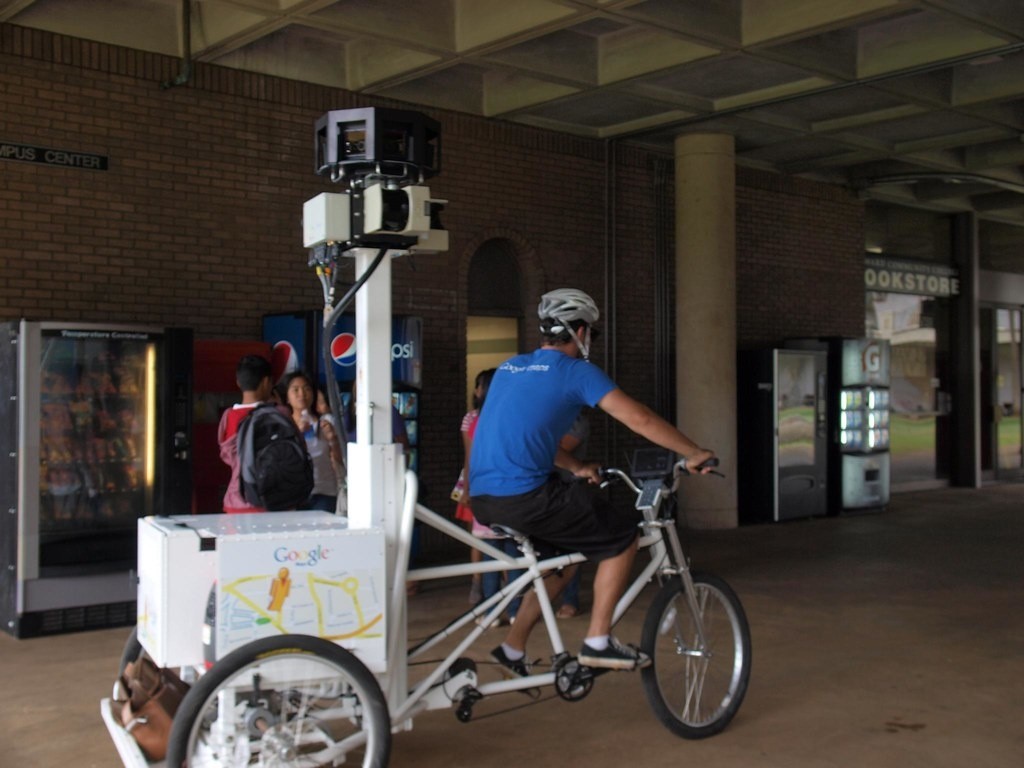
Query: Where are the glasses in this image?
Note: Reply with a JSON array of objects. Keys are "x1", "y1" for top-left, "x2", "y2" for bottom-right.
[{"x1": 539, "y1": 324, "x2": 582, "y2": 335}]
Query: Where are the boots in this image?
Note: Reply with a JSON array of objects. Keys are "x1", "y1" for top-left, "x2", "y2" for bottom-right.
[{"x1": 469, "y1": 577, "x2": 481, "y2": 604}]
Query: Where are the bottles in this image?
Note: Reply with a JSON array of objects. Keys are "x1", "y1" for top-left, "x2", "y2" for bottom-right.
[{"x1": 299, "y1": 409, "x2": 321, "y2": 458}]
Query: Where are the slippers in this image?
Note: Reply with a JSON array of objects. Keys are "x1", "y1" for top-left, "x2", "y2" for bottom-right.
[{"x1": 555, "y1": 604, "x2": 575, "y2": 620}]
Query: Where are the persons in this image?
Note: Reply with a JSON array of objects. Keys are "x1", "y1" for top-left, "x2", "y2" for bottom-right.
[
  {"x1": 450, "y1": 368, "x2": 590, "y2": 628},
  {"x1": 466, "y1": 288, "x2": 717, "y2": 700},
  {"x1": 218, "y1": 353, "x2": 426, "y2": 596}
]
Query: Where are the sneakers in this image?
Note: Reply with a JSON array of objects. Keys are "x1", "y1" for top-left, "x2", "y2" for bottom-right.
[
  {"x1": 489, "y1": 645, "x2": 542, "y2": 699},
  {"x1": 509, "y1": 615, "x2": 517, "y2": 626},
  {"x1": 473, "y1": 614, "x2": 501, "y2": 628},
  {"x1": 576, "y1": 635, "x2": 652, "y2": 671}
]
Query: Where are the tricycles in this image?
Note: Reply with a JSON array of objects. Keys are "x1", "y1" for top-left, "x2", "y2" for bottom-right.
[{"x1": 99, "y1": 455, "x2": 752, "y2": 768}]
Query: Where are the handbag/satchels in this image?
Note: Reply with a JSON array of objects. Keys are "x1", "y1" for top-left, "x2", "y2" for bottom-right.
[{"x1": 119, "y1": 653, "x2": 192, "y2": 761}]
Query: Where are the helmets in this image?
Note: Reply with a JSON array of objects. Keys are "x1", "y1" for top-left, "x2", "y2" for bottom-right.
[{"x1": 536, "y1": 285, "x2": 600, "y2": 327}]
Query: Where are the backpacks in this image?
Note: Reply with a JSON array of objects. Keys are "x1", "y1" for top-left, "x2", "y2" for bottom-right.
[{"x1": 236, "y1": 401, "x2": 315, "y2": 511}]
